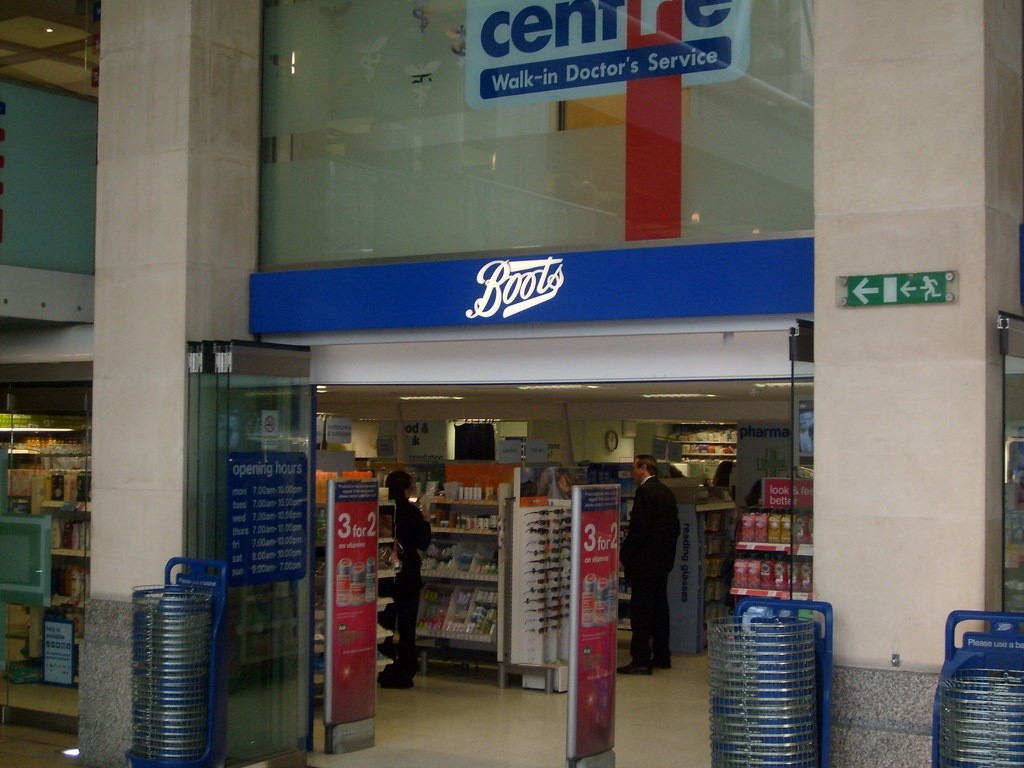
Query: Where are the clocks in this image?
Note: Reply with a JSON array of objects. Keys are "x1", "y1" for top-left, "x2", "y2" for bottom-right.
[{"x1": 606, "y1": 429, "x2": 618, "y2": 450}]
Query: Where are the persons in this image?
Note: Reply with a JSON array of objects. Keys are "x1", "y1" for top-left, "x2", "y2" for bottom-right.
[
  {"x1": 713, "y1": 461, "x2": 734, "y2": 487},
  {"x1": 377, "y1": 471, "x2": 431, "y2": 688},
  {"x1": 616, "y1": 455, "x2": 680, "y2": 675},
  {"x1": 746, "y1": 479, "x2": 761, "y2": 505}
]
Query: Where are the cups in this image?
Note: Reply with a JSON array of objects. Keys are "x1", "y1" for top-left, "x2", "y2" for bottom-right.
[
  {"x1": 378, "y1": 487, "x2": 389, "y2": 505},
  {"x1": 415, "y1": 480, "x2": 462, "y2": 500}
]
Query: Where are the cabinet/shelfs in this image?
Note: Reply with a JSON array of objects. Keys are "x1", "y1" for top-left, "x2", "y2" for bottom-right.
[{"x1": 0, "y1": 425, "x2": 814, "y2": 693}]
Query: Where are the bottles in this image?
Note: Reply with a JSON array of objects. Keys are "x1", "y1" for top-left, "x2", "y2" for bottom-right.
[
  {"x1": 51, "y1": 518, "x2": 89, "y2": 550},
  {"x1": 458, "y1": 483, "x2": 494, "y2": 501},
  {"x1": 1, "y1": 435, "x2": 80, "y2": 452},
  {"x1": 52, "y1": 563, "x2": 89, "y2": 639},
  {"x1": 315, "y1": 470, "x2": 373, "y2": 503},
  {"x1": 29, "y1": 469, "x2": 90, "y2": 515},
  {"x1": 418, "y1": 504, "x2": 497, "y2": 531}
]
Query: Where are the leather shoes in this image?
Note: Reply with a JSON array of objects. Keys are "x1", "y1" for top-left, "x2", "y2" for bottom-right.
[
  {"x1": 616, "y1": 661, "x2": 652, "y2": 675},
  {"x1": 651, "y1": 659, "x2": 671, "y2": 669}
]
